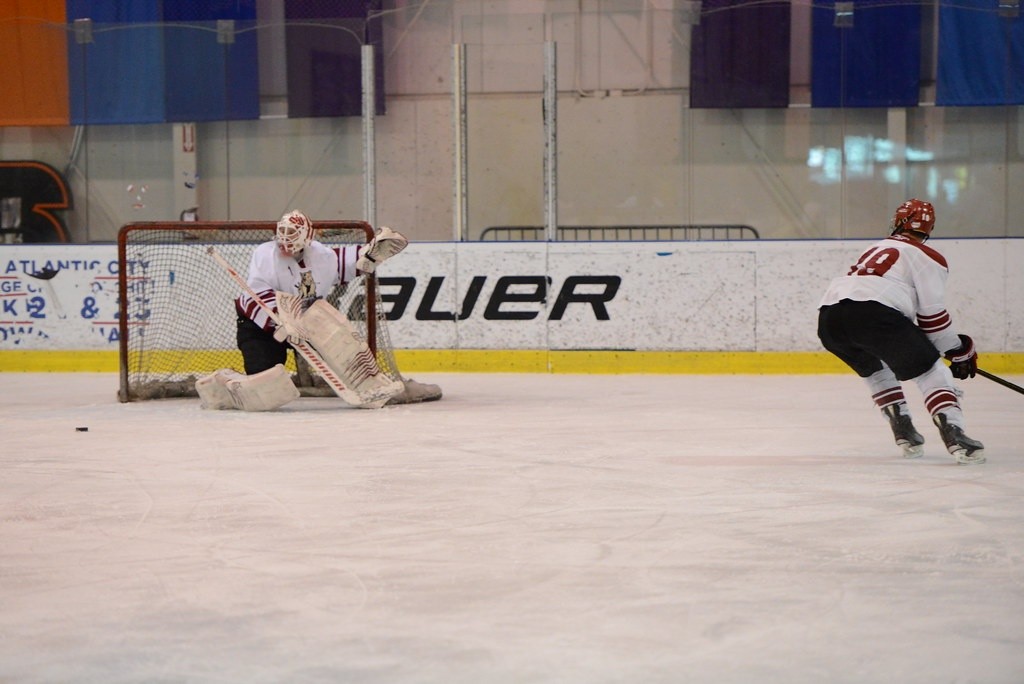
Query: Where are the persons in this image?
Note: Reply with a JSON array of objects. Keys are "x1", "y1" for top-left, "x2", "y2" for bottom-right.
[
  {"x1": 233, "y1": 208, "x2": 339, "y2": 375},
  {"x1": 815, "y1": 198, "x2": 985, "y2": 464}
]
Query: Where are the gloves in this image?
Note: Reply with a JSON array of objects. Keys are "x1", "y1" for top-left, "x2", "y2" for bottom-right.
[{"x1": 945, "y1": 334, "x2": 977, "y2": 379}]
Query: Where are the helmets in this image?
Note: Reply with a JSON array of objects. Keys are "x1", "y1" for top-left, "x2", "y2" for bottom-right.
[
  {"x1": 894, "y1": 199, "x2": 935, "y2": 236},
  {"x1": 276, "y1": 209, "x2": 313, "y2": 256}
]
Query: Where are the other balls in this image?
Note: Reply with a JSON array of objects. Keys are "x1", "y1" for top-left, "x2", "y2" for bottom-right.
[{"x1": 77, "y1": 428, "x2": 89, "y2": 432}]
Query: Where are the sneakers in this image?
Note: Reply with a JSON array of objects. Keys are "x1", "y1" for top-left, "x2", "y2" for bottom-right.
[
  {"x1": 891, "y1": 415, "x2": 925, "y2": 460},
  {"x1": 941, "y1": 424, "x2": 985, "y2": 464}
]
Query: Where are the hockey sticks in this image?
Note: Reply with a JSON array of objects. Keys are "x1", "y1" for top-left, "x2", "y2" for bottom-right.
[
  {"x1": 976, "y1": 369, "x2": 1024, "y2": 394},
  {"x1": 204, "y1": 245, "x2": 404, "y2": 408}
]
